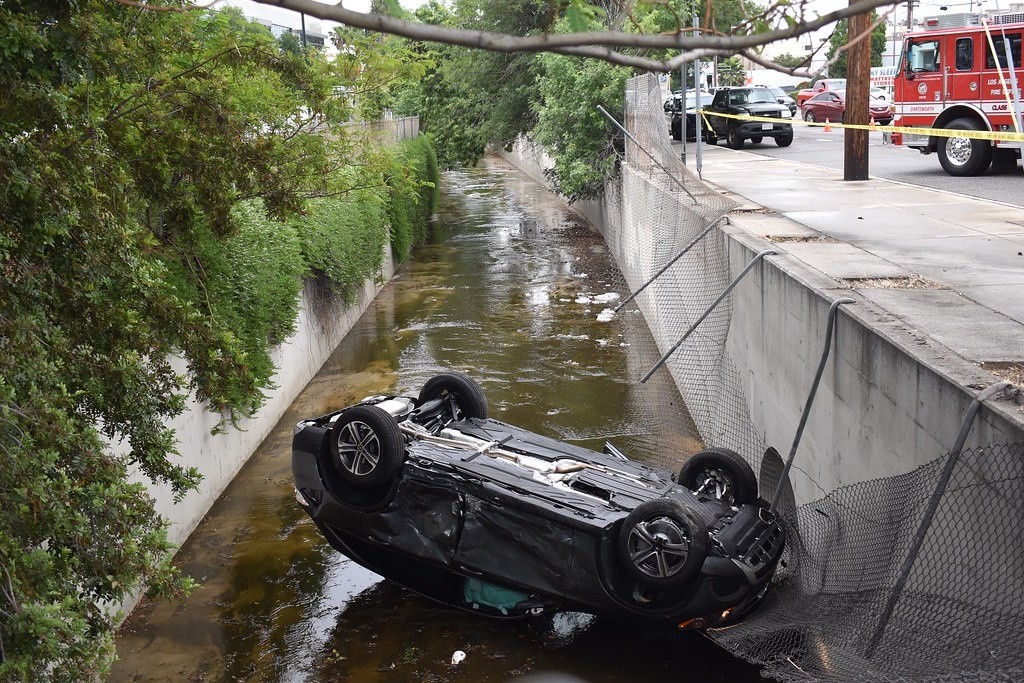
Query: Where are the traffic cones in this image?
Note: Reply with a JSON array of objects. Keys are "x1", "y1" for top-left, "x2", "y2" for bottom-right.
[
  {"x1": 868, "y1": 116, "x2": 878, "y2": 132},
  {"x1": 822, "y1": 116, "x2": 833, "y2": 133}
]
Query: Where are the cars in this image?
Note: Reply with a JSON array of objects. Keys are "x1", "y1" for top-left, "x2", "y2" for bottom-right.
[
  {"x1": 664, "y1": 85, "x2": 747, "y2": 112},
  {"x1": 291, "y1": 370, "x2": 790, "y2": 639},
  {"x1": 766, "y1": 86, "x2": 796, "y2": 117},
  {"x1": 671, "y1": 91, "x2": 715, "y2": 142},
  {"x1": 801, "y1": 88, "x2": 895, "y2": 126}
]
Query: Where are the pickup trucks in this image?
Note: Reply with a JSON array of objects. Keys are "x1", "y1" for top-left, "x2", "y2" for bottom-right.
[
  {"x1": 702, "y1": 85, "x2": 793, "y2": 150},
  {"x1": 796, "y1": 78, "x2": 847, "y2": 109}
]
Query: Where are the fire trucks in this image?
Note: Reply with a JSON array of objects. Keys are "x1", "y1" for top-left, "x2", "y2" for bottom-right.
[{"x1": 882, "y1": 3, "x2": 1024, "y2": 177}]
